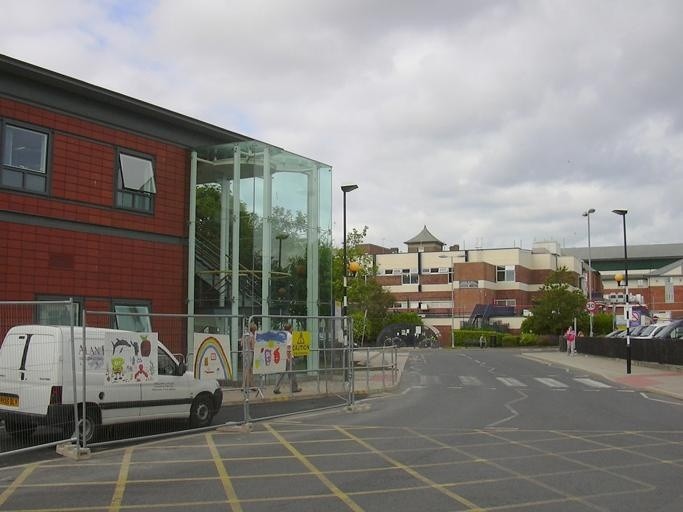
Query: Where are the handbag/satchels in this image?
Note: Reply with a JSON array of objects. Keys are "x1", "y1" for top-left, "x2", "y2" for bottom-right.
[{"x1": 563, "y1": 334, "x2": 574, "y2": 340}]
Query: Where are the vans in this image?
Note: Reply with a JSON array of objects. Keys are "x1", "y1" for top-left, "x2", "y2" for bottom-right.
[{"x1": 0, "y1": 324, "x2": 223, "y2": 445}]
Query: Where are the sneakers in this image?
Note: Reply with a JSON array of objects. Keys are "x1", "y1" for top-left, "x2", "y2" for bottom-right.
[
  {"x1": 292, "y1": 388, "x2": 302, "y2": 393},
  {"x1": 273, "y1": 389, "x2": 281, "y2": 394}
]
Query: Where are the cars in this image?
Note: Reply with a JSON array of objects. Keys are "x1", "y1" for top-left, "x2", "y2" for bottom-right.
[{"x1": 605, "y1": 320, "x2": 683, "y2": 339}]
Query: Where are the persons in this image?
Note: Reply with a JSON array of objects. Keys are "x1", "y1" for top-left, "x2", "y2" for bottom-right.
[
  {"x1": 241, "y1": 322, "x2": 256, "y2": 391},
  {"x1": 273, "y1": 323, "x2": 302, "y2": 394},
  {"x1": 565, "y1": 325, "x2": 576, "y2": 357}
]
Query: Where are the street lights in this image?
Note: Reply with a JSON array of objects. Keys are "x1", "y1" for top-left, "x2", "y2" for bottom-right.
[
  {"x1": 582, "y1": 209, "x2": 595, "y2": 337},
  {"x1": 276, "y1": 234, "x2": 290, "y2": 270},
  {"x1": 612, "y1": 209, "x2": 631, "y2": 374},
  {"x1": 341, "y1": 184, "x2": 358, "y2": 314},
  {"x1": 439, "y1": 254, "x2": 467, "y2": 348},
  {"x1": 551, "y1": 253, "x2": 561, "y2": 271}
]
